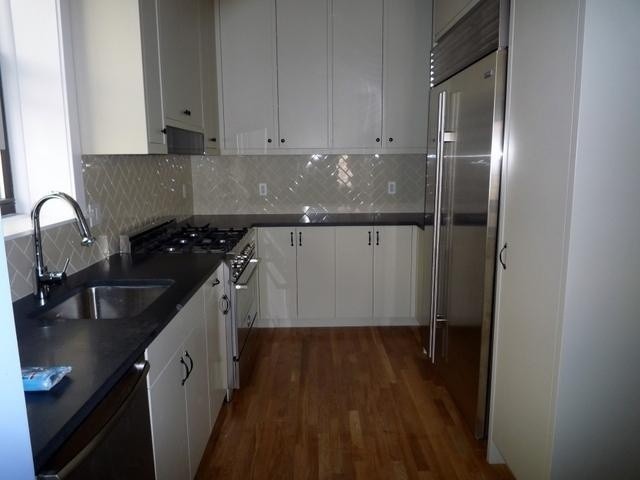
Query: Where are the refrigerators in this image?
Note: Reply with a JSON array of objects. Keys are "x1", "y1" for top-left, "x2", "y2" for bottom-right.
[{"x1": 416, "y1": 1, "x2": 511, "y2": 440}]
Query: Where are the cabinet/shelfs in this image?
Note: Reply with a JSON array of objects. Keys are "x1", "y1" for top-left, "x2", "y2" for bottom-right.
[
  {"x1": 335, "y1": 226, "x2": 413, "y2": 326},
  {"x1": 204, "y1": 260, "x2": 229, "y2": 434},
  {"x1": 329, "y1": 1, "x2": 432, "y2": 153},
  {"x1": 217, "y1": 0, "x2": 329, "y2": 154},
  {"x1": 70, "y1": 1, "x2": 221, "y2": 158},
  {"x1": 144, "y1": 282, "x2": 212, "y2": 477},
  {"x1": 254, "y1": 227, "x2": 337, "y2": 326}
]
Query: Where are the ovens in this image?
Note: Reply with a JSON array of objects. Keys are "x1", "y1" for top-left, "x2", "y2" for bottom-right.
[{"x1": 231, "y1": 242, "x2": 262, "y2": 392}]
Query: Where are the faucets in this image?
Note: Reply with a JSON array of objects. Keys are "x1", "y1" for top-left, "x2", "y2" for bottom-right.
[{"x1": 30, "y1": 192, "x2": 96, "y2": 299}]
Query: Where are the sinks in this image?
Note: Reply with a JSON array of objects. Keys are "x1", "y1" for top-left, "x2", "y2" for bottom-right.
[{"x1": 26, "y1": 278, "x2": 170, "y2": 320}]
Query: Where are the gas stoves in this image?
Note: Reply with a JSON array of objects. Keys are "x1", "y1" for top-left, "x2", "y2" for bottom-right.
[{"x1": 131, "y1": 222, "x2": 256, "y2": 291}]
